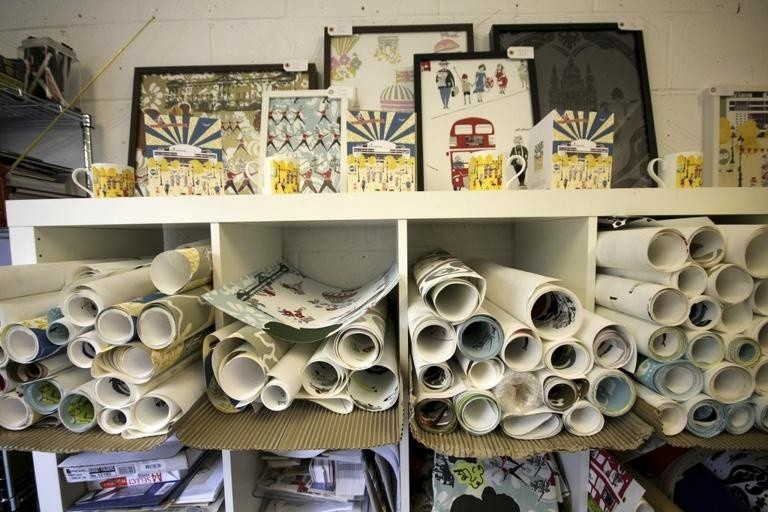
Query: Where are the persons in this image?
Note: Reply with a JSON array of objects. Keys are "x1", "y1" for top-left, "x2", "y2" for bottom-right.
[
  {"x1": 221, "y1": 105, "x2": 341, "y2": 194},
  {"x1": 510, "y1": 135, "x2": 528, "y2": 186},
  {"x1": 436, "y1": 59, "x2": 528, "y2": 109}
]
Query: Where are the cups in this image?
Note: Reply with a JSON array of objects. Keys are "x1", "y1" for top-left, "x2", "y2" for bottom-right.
[
  {"x1": 72, "y1": 163, "x2": 135, "y2": 198},
  {"x1": 244, "y1": 156, "x2": 301, "y2": 196},
  {"x1": 647, "y1": 152, "x2": 705, "y2": 188},
  {"x1": 468, "y1": 150, "x2": 526, "y2": 191}
]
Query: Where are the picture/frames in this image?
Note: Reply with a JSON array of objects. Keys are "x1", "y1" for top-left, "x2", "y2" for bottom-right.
[
  {"x1": 257, "y1": 87, "x2": 346, "y2": 192},
  {"x1": 323, "y1": 24, "x2": 475, "y2": 159},
  {"x1": 127, "y1": 63, "x2": 319, "y2": 172},
  {"x1": 488, "y1": 23, "x2": 660, "y2": 189},
  {"x1": 413, "y1": 50, "x2": 541, "y2": 191}
]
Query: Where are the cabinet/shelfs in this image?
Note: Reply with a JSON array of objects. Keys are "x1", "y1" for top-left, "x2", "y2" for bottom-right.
[{"x1": 0, "y1": 182, "x2": 768, "y2": 512}]
